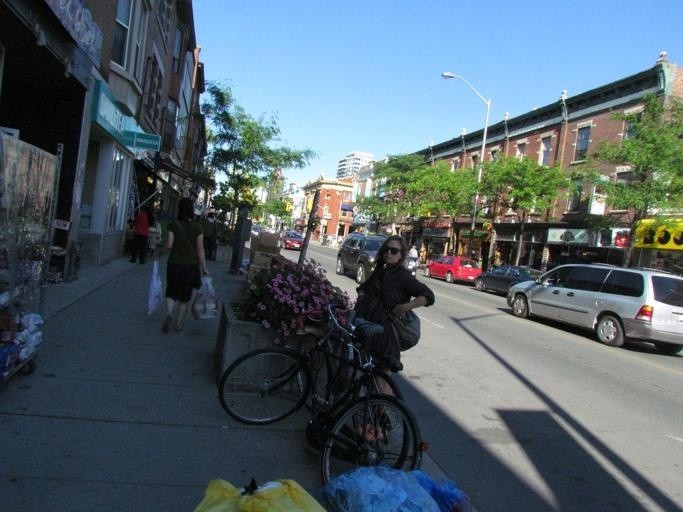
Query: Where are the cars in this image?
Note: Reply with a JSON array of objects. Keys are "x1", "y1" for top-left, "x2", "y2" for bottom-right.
[
  {"x1": 423, "y1": 255, "x2": 543, "y2": 296},
  {"x1": 250, "y1": 224, "x2": 304, "y2": 250}
]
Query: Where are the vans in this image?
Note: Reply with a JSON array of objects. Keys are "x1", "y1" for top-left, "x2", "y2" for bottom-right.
[{"x1": 506, "y1": 262, "x2": 682, "y2": 355}]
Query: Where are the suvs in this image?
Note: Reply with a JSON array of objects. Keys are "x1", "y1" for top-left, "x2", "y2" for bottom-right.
[{"x1": 335, "y1": 232, "x2": 417, "y2": 285}]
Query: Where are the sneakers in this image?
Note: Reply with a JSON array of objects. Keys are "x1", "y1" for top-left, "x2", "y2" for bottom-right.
[{"x1": 164, "y1": 312, "x2": 183, "y2": 333}]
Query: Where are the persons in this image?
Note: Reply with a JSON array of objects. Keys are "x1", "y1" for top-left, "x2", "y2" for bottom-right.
[
  {"x1": 408, "y1": 245, "x2": 418, "y2": 261},
  {"x1": 126, "y1": 198, "x2": 218, "y2": 333},
  {"x1": 352, "y1": 236, "x2": 435, "y2": 442}
]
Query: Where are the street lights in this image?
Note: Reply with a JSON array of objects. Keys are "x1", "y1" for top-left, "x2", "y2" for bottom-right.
[{"x1": 442, "y1": 71, "x2": 491, "y2": 257}]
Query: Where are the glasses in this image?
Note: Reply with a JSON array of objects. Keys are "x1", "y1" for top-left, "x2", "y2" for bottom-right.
[{"x1": 382, "y1": 245, "x2": 401, "y2": 255}]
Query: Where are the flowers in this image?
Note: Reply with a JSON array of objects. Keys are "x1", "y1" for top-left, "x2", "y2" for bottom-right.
[{"x1": 241, "y1": 259, "x2": 353, "y2": 347}]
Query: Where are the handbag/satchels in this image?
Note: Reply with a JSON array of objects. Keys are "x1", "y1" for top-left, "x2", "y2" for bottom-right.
[{"x1": 382, "y1": 309, "x2": 421, "y2": 352}]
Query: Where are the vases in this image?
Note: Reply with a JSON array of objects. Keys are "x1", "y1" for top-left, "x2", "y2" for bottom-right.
[{"x1": 215, "y1": 301, "x2": 332, "y2": 395}]
